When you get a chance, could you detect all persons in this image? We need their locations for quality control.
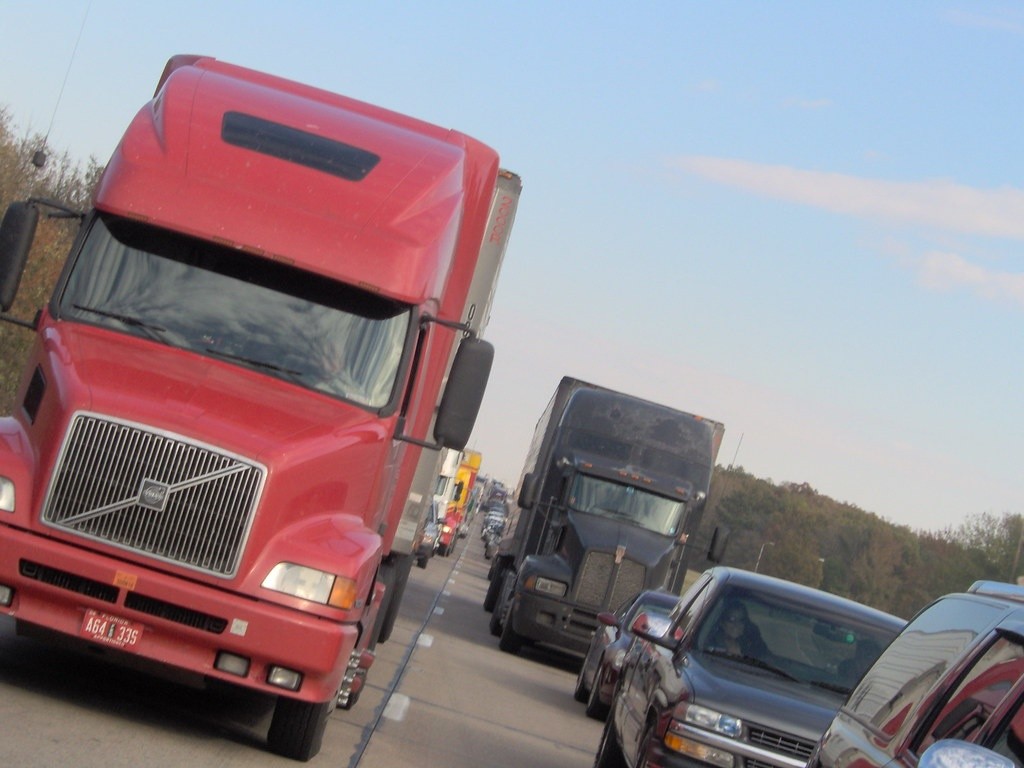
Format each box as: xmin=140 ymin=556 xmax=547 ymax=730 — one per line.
xmin=704 ymin=602 xmax=757 ymax=660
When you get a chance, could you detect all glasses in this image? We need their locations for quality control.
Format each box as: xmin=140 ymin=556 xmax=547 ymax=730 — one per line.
xmin=728 ymin=615 xmax=747 ymax=623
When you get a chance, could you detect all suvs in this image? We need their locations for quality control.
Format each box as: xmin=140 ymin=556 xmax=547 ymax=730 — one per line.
xmin=801 ymin=581 xmax=1024 ymax=768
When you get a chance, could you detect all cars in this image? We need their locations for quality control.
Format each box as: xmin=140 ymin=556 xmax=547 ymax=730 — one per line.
xmin=590 ymin=565 xmax=909 ymax=768
xmin=415 ymin=446 xmax=514 ymax=572
xmin=572 ymin=590 xmax=685 ymax=722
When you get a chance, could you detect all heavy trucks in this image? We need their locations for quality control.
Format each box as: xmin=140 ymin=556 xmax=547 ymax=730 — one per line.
xmin=484 ymin=376 xmax=730 ymax=661
xmin=0 ymin=53 xmax=522 ymax=763
